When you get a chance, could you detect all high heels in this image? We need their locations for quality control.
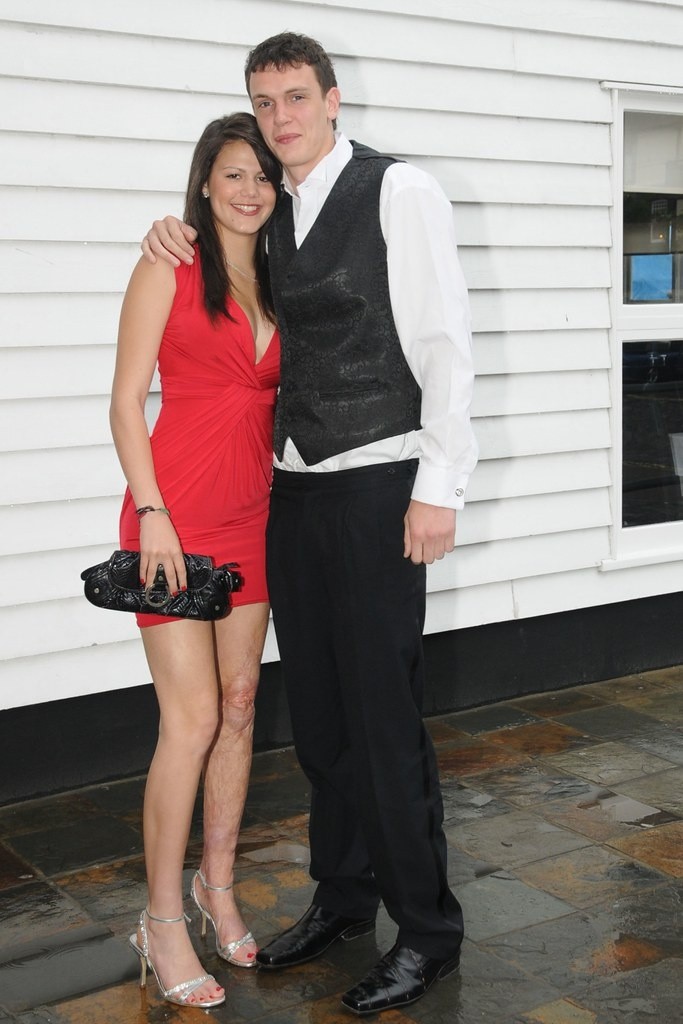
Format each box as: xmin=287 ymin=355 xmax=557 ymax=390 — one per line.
xmin=129 ymin=905 xmax=225 ymax=1008
xmin=189 ymin=868 xmax=261 ymax=968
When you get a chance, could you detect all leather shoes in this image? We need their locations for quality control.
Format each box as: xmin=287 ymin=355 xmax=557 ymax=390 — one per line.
xmin=340 ymin=941 xmax=462 ymax=1015
xmin=255 ymin=904 xmax=377 ymax=969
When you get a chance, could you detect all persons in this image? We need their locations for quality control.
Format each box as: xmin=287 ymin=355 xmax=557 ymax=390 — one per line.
xmin=109 ymin=32 xmax=479 ymax=1014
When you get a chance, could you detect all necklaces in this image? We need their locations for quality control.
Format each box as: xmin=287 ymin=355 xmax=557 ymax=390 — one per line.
xmin=222 ymin=256 xmax=258 ymax=282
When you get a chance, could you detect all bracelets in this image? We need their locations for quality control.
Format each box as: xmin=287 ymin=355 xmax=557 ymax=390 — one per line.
xmin=135 ymin=506 xmax=170 ymax=524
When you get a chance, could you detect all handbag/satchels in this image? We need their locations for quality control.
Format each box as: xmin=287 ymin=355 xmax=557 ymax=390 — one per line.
xmin=80 ymin=547 xmax=242 ymax=622
